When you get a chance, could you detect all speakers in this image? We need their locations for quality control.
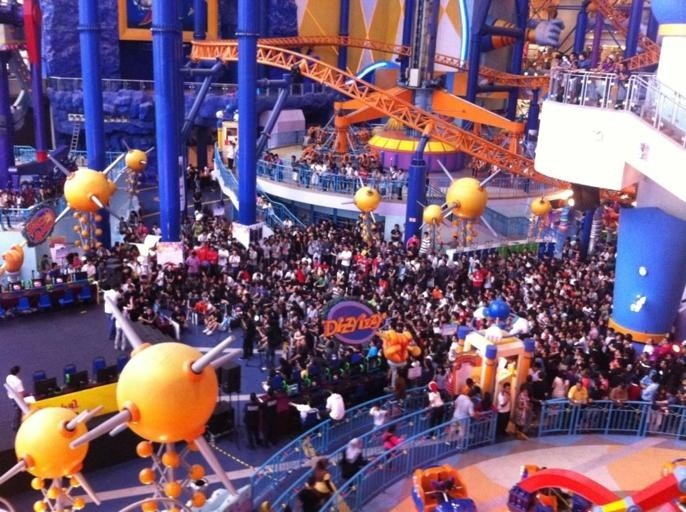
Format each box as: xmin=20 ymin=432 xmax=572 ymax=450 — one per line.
xmin=209 ymin=401 xmax=235 ymax=435
xmin=220 ymin=362 xmax=240 ymax=394
xmin=104 ymin=258 xmax=123 ymax=288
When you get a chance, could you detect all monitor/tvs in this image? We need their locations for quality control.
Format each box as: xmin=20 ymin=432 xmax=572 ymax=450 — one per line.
xmin=70 ymin=370 xmax=88 ymax=385
xmin=36 ymin=377 xmax=57 ymax=393
xmin=97 ymin=365 xmax=117 ymax=381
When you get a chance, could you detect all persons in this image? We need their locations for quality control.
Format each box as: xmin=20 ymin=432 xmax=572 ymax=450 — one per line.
xmin=41 ymin=245 xmax=111 ymax=286
xmin=535 ymin=45 xmax=630 ymax=108
xmin=0 ymin=172 xmax=65 ymax=233
xmin=6 ymin=366 xmax=24 ymax=430
xmin=104 ymin=130 xmax=686 ymax=512
